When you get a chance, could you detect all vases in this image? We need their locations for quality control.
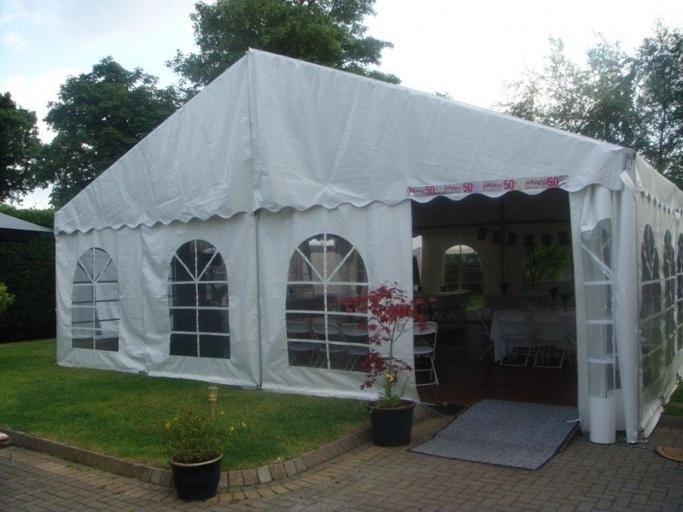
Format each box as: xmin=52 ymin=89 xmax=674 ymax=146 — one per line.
xmin=169 ymin=454 xmax=223 ymax=502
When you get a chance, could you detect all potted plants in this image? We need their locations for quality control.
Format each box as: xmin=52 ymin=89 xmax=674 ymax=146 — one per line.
xmin=342 ymin=281 xmax=437 ymax=447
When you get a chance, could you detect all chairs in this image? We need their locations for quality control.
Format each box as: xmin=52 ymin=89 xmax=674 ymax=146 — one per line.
xmin=287 ymin=320 xmax=438 ymax=388
xmin=476 ymin=306 xmax=569 ymax=371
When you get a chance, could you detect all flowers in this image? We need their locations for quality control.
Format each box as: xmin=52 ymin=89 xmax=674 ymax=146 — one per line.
xmin=165 ymin=403 xmax=245 ymax=460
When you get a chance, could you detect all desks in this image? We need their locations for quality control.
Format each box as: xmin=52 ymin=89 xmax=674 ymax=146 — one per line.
xmin=413 ymin=290 xmax=471 ymax=324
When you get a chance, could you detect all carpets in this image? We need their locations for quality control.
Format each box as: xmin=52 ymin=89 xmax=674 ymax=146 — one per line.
xmin=407 ymin=399 xmax=579 ymax=471
